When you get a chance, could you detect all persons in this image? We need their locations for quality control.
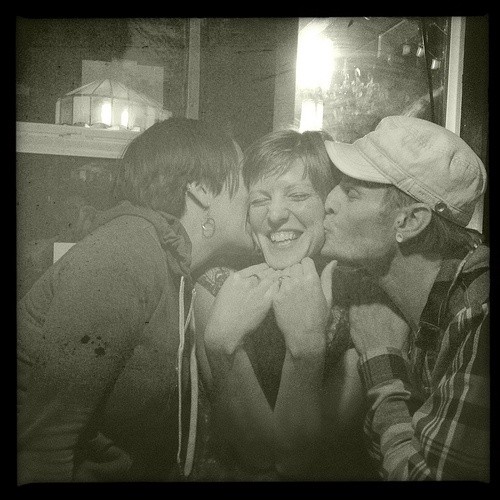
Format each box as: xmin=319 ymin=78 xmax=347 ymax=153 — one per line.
xmin=320 ymin=115 xmax=490 ymax=481
xmin=15 ymin=118 xmax=250 ymax=481
xmin=194 ymin=129 xmax=357 ymax=481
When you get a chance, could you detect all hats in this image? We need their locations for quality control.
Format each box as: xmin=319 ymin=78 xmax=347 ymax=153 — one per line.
xmin=325 ymin=116 xmax=486 ymax=232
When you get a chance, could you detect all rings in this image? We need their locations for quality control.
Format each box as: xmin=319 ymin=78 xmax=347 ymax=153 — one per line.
xmin=278 ymin=274 xmax=292 ymax=283
xmin=248 ymin=274 xmax=261 ymax=286
